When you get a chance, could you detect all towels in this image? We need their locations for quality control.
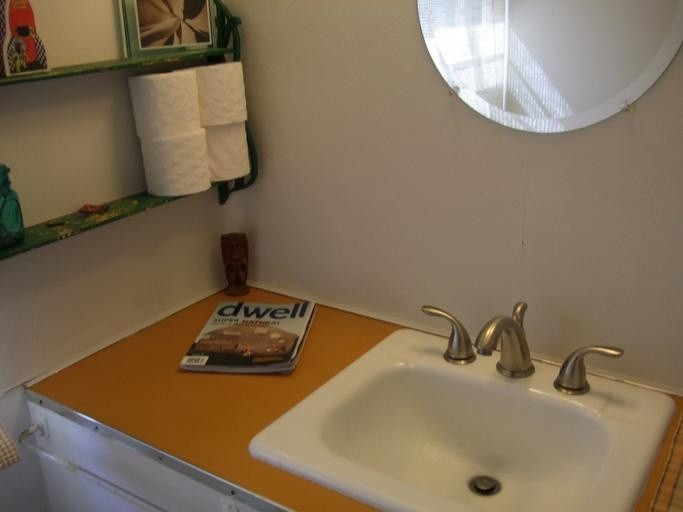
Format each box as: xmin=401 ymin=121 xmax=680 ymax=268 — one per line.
xmin=0 ymin=419 xmax=20 ymax=472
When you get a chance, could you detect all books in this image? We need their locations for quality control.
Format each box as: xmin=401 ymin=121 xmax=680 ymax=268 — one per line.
xmin=177 ymin=300 xmax=319 ymax=377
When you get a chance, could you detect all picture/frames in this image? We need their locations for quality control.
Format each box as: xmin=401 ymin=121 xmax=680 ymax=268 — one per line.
xmin=122 ymin=0 xmax=217 ymax=58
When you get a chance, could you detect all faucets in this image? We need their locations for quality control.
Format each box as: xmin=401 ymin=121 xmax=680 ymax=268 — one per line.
xmin=474 ymin=303 xmax=534 ymax=378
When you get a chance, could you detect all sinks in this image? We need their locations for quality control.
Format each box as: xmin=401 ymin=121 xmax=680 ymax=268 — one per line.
xmin=248 ymin=328 xmax=677 ymax=512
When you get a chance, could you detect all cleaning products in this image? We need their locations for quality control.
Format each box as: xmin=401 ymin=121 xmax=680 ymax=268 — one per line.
xmin=0 ymin=163 xmax=25 ymax=251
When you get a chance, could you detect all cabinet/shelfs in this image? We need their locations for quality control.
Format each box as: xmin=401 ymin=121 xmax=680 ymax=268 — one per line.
xmin=0 ymin=0 xmax=234 ymax=261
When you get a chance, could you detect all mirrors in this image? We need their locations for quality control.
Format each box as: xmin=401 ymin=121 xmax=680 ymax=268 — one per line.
xmin=415 ymin=0 xmax=683 ymax=133
